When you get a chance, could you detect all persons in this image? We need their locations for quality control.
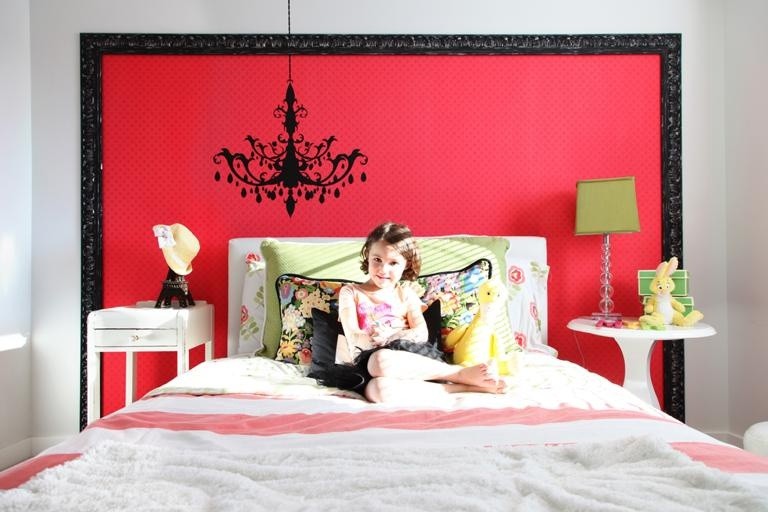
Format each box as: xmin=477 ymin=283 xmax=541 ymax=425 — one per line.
xmin=337 ymin=221 xmax=510 ymax=406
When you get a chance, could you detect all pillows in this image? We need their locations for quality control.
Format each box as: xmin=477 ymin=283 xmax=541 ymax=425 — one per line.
xmin=238 ymin=235 xmax=558 ymax=363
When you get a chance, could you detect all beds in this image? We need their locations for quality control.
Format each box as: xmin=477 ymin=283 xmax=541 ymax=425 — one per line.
xmin=0 ymin=234 xmax=767 ymax=512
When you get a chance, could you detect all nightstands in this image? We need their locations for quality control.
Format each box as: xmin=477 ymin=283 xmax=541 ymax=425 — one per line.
xmin=87 ymin=305 xmax=215 ymax=424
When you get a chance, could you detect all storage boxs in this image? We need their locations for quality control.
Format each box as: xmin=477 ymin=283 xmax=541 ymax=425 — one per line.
xmin=637 ymin=270 xmax=694 ymax=316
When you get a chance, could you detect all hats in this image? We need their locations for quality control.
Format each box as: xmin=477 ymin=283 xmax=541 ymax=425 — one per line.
xmin=153 ymin=223 xmax=201 ymax=276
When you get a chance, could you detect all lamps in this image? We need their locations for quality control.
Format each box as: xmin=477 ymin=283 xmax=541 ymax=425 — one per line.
xmin=575 ymin=176 xmax=640 ymax=321
